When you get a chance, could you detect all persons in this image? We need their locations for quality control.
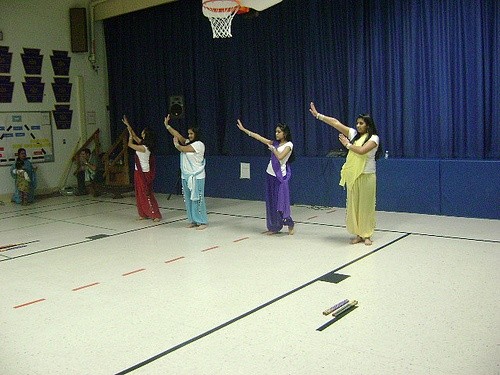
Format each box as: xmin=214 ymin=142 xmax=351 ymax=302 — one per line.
xmin=122 ymin=115 xmax=162 ymax=222
xmin=73 ymin=148 xmax=101 ymax=197
xmin=164 ymin=114 xmax=208 ymax=230
xmin=10 ymin=148 xmax=37 ymax=206
xmin=309 ymin=102 xmax=383 ymax=246
xmin=237 ymin=118 xmax=296 ymax=235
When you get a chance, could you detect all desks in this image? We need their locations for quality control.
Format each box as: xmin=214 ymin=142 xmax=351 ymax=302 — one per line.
xmin=152 ymin=155 xmax=500 ymax=220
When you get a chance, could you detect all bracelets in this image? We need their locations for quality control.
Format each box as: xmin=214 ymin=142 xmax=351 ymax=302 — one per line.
xmin=345 ymin=143 xmax=352 ymax=149
xmin=270 ymin=147 xmax=275 ymax=151
xmin=166 ymin=126 xmax=171 ymax=129
xmin=129 ymin=137 xmax=132 ymax=139
xmin=249 ymin=131 xmax=250 ymax=136
xmin=316 ymin=113 xmax=324 ymax=120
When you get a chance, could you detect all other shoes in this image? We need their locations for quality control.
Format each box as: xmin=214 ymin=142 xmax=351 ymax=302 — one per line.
xmin=76 ymin=190 xmax=88 ymax=196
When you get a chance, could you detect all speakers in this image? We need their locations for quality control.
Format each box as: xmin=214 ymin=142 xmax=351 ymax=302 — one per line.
xmin=69 ymin=8 xmax=88 ymax=52
xmin=168 ymin=96 xmax=185 ymax=119
